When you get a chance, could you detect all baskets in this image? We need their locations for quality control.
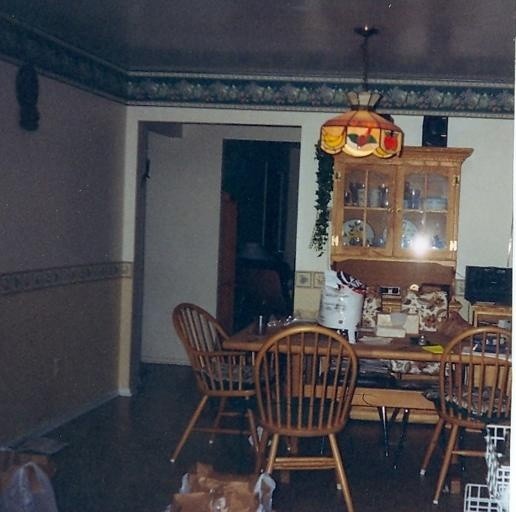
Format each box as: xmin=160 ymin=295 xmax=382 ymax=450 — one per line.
xmin=484 ymin=423 xmax=510 ymax=511
xmin=464 ymin=483 xmax=499 ymax=512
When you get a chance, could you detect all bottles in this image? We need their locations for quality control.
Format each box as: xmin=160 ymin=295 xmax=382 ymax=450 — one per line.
xmin=344 ymin=181 xmax=422 ymax=209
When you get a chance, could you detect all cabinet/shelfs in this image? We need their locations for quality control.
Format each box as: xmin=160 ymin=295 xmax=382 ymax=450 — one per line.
xmin=328 ymin=147 xmax=475 ymax=272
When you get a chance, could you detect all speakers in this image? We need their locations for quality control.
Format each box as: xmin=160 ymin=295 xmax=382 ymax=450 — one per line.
xmin=421 ymin=114 xmax=448 ymax=147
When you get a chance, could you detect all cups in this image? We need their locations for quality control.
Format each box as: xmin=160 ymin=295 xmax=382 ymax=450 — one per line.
xmin=256 ymin=315 xmax=267 ymax=336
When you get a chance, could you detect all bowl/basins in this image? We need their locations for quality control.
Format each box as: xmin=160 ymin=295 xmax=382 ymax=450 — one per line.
xmin=423 ymin=198 xmax=448 ymax=211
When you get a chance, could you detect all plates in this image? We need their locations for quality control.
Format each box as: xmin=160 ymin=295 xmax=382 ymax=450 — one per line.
xmin=341 ymin=218 xmax=376 ymax=248
xmin=382 ymin=219 xmax=419 ymax=252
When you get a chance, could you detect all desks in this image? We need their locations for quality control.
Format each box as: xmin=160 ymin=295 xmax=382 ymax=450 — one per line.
xmin=222 ymin=318 xmax=512 ymax=484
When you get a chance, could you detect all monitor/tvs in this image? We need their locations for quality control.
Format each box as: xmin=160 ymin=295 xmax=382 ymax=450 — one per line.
xmin=462 ymin=264 xmax=511 ymax=305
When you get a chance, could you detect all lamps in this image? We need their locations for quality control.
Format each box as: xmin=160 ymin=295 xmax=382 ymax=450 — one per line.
xmin=319 ymin=26 xmax=404 ymax=159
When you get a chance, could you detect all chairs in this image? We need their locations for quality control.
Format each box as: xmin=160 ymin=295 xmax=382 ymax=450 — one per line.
xmin=251 ymin=321 xmax=360 ymax=512
xmin=419 ymin=325 xmax=511 ymax=505
xmin=469 ymin=303 xmax=511 ymax=330
xmin=169 ymin=302 xmax=274 ymax=473
